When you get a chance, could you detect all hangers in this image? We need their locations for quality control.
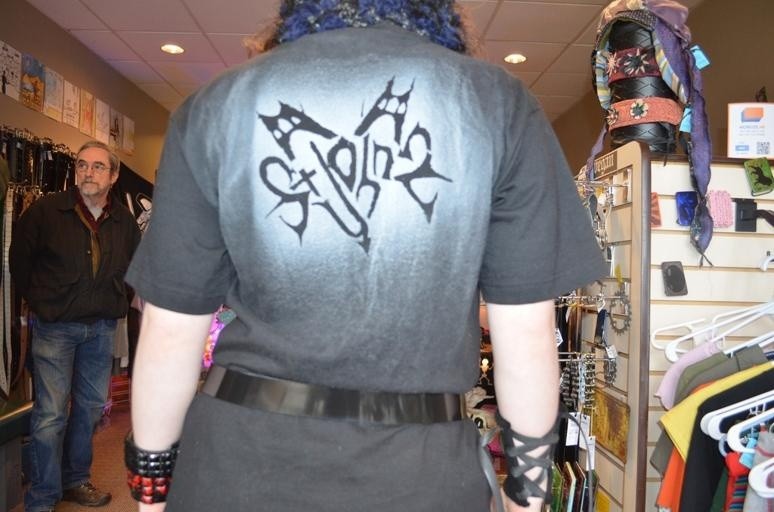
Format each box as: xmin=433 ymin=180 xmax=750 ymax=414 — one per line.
xmin=651 ymin=254 xmax=774 ymax=500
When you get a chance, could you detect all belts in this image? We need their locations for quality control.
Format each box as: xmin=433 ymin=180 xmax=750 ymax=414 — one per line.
xmin=202 ymin=360 xmax=468 ymax=424
xmin=0 ymin=130 xmax=77 ymax=399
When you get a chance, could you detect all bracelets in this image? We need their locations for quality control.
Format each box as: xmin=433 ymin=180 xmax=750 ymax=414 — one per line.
xmin=475 ymin=397 xmax=570 ymax=507
xmin=124 ymin=428 xmax=180 ymax=506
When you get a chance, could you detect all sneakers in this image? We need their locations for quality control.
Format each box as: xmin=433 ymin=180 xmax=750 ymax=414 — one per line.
xmin=67 ymin=483 xmax=111 ymax=508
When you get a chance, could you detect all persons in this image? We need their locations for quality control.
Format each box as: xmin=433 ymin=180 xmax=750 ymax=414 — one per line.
xmin=8 ymin=140 xmax=141 ymax=511
xmin=121 ymin=0 xmax=609 ymax=512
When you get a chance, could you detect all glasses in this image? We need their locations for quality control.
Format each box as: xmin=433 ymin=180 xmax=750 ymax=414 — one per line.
xmin=73 ymin=161 xmax=114 ymax=174
